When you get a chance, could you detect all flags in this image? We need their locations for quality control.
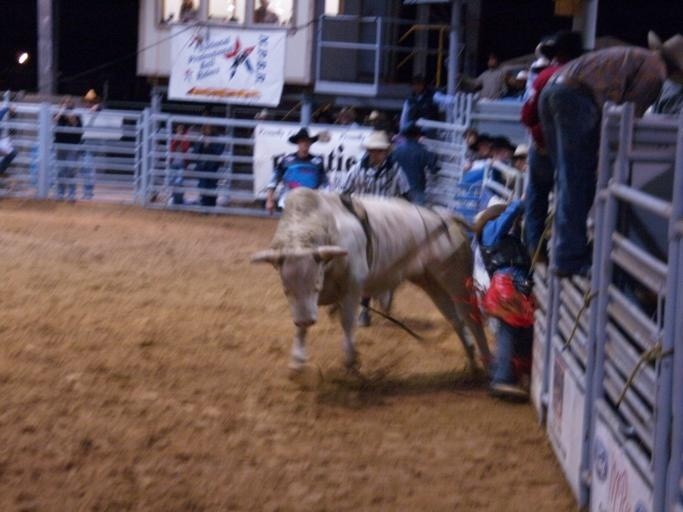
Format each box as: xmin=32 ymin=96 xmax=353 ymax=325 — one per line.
xmin=166 ymin=20 xmax=289 ymax=110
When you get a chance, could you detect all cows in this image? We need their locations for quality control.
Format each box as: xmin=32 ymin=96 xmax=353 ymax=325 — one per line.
xmin=249 ymin=186 xmax=508 ymax=391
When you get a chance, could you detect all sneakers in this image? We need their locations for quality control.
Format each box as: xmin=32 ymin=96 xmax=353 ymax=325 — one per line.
xmin=527 ymin=242 xmax=593 ymax=279
xmin=486 ymin=379 xmax=535 ymax=402
xmin=357 ymin=306 xmax=373 ymax=328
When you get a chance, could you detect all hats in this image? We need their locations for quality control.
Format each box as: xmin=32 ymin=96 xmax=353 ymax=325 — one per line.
xmin=646 ymin=31 xmax=682 ymax=85
xmin=362 ymin=109 xmax=388 ymax=123
xmin=512 ymin=144 xmax=531 ymax=160
xmin=80 ymin=89 xmax=102 ymax=103
xmin=467 ymin=133 xmax=496 ymax=151
xmin=287 ymin=127 xmax=320 ymax=146
xmin=361 ymin=130 xmax=394 ymax=151
xmin=398 ymin=123 xmax=427 ymax=136
xmin=471 ymin=193 xmax=509 ymax=229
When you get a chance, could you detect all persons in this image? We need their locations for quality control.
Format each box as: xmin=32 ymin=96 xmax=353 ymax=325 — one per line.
xmin=178 ymin=0 xmax=199 ymax=21
xmin=252 ymin=0 xmax=279 ymax=24
xmin=471 ymin=191 xmax=540 ymax=403
xmin=192 ymin=107 xmax=226 ymax=206
xmin=47 ymin=93 xmax=85 ymax=205
xmin=72 ymin=87 xmax=104 ymax=201
xmin=163 ymin=120 xmax=195 ymax=204
xmin=0 ymin=86 xmax=28 ymax=175
